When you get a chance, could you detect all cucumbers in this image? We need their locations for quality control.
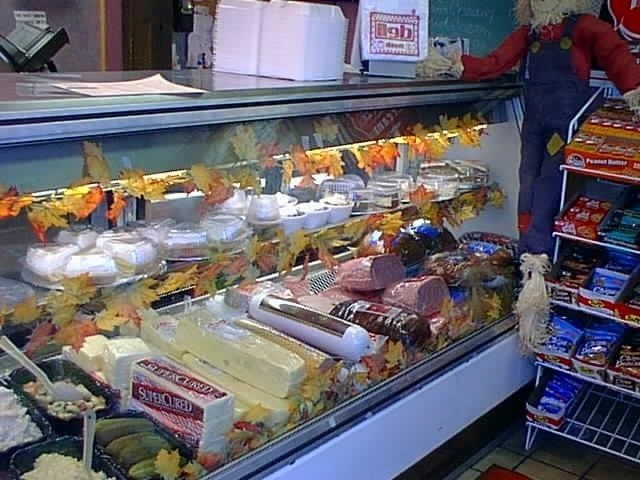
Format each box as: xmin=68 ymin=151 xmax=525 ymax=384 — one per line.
xmin=95 ymin=417 xmax=186 ymax=480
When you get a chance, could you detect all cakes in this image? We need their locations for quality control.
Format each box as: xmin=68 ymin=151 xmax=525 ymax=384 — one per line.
xmin=325 ymin=164 xmax=486 ymax=213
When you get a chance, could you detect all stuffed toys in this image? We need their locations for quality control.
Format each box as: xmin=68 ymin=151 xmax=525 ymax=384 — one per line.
xmin=413 ymin=0 xmax=639 ymax=353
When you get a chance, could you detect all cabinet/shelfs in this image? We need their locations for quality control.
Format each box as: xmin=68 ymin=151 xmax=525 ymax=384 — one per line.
xmin=2 ymin=68 xmax=541 ymax=478
xmin=524 ymin=85 xmax=640 ymax=462
